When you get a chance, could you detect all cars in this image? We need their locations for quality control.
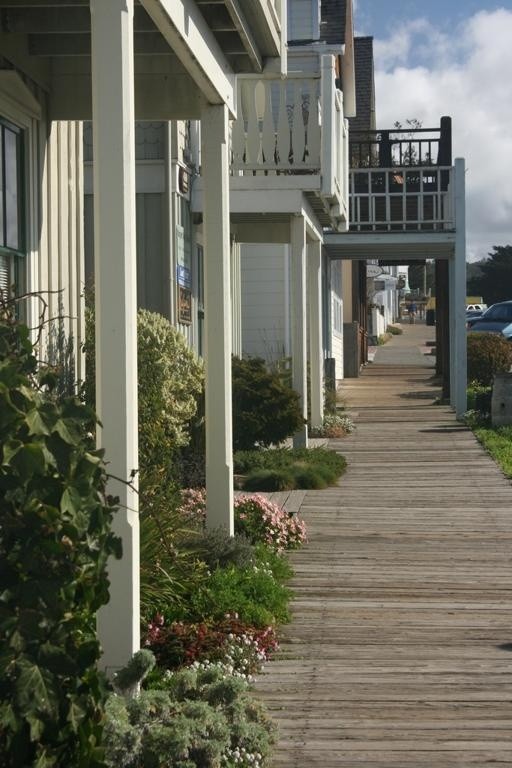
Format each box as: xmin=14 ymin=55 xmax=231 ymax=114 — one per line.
xmin=466 ymin=300 xmax=512 ymax=341
